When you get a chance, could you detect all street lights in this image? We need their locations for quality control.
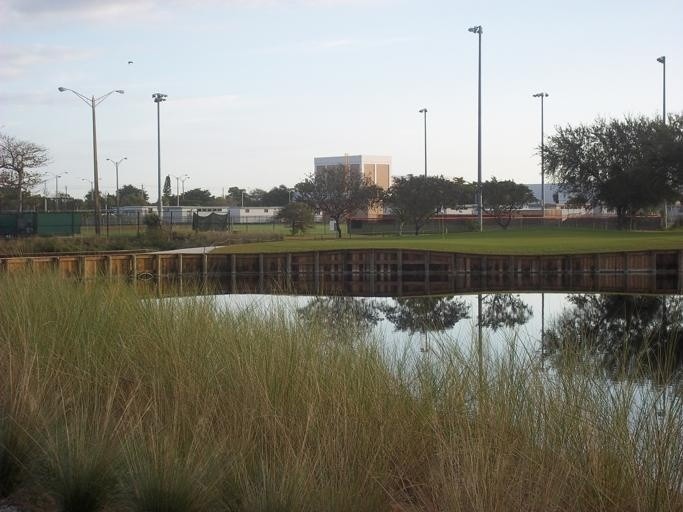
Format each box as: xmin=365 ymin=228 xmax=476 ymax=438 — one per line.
xmin=418 ymin=108 xmax=429 ymax=179
xmin=531 ymin=92 xmax=551 ymax=209
xmin=467 ymin=23 xmax=486 ymax=232
xmin=151 ymin=90 xmax=170 ymax=222
xmin=46 ymin=170 xmax=69 ymax=209
xmin=169 ymin=172 xmax=188 ymax=206
xmin=654 ymin=55 xmax=666 ymax=125
xmin=104 ymin=156 xmax=127 ymax=207
xmin=55 ymin=85 xmax=126 ymax=236
xmin=80 ymin=175 xmax=102 ymax=208
